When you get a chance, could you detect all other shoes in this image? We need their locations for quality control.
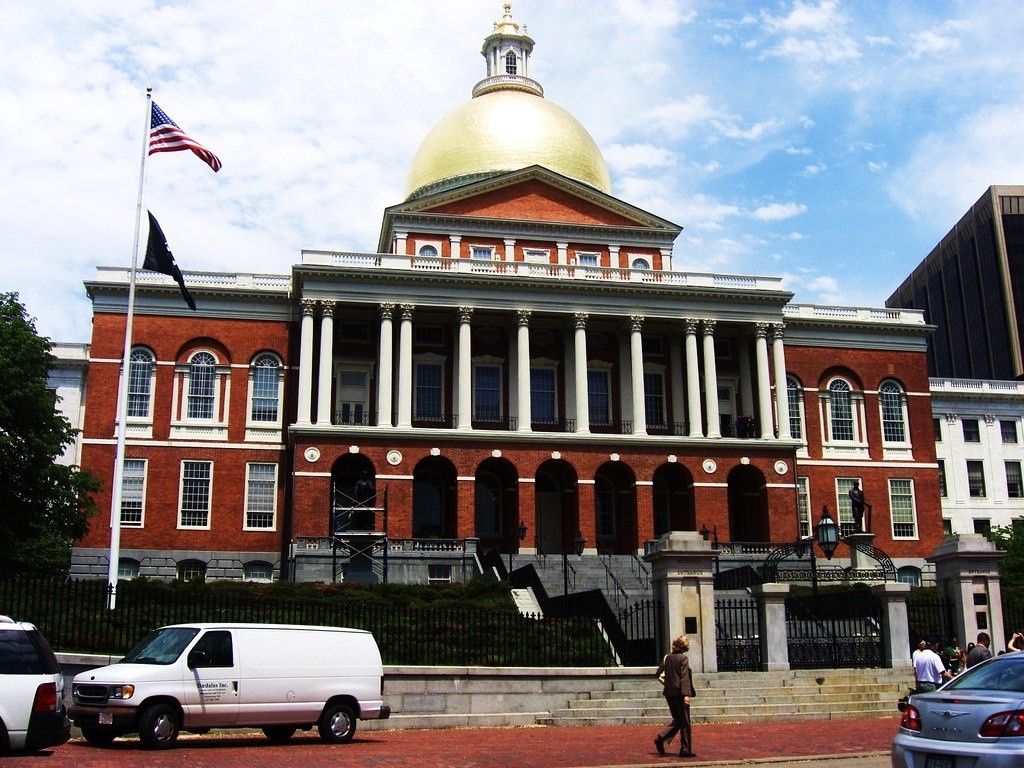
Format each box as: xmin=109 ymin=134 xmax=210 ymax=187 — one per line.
xmin=654 ymin=736 xmax=667 ymax=754
xmin=681 ymin=752 xmax=697 ymax=758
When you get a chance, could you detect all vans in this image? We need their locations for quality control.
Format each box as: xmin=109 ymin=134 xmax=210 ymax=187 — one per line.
xmin=66 ymin=622 xmax=393 ymax=752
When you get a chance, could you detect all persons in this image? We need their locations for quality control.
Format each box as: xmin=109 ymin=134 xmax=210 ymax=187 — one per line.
xmin=654 ymin=635 xmax=697 ymax=756
xmin=911 ymin=632 xmax=1024 ymax=692
xmin=849 ymin=481 xmax=871 ymax=532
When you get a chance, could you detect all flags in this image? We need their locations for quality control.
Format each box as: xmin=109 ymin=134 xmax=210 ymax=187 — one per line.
xmin=148 ymin=101 xmax=222 ymax=172
xmin=142 ymin=210 xmax=196 ymax=312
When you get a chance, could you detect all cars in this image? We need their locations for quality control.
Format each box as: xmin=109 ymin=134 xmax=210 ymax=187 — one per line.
xmin=0 ymin=613 xmax=71 ymax=755
xmin=891 ymin=646 xmax=1024 ymax=768
